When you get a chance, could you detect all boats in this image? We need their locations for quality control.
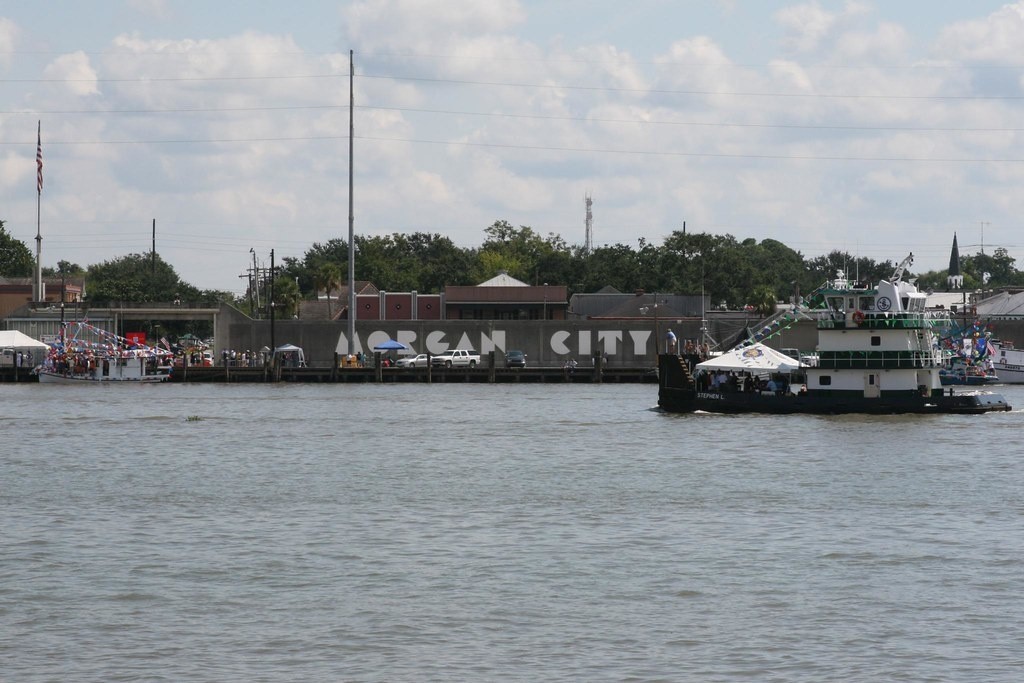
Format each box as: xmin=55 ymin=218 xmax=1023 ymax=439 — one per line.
xmin=657 ymin=252 xmax=1013 ymax=415
xmin=937 ymin=292 xmax=1000 ymax=385
xmin=38 ymin=311 xmax=175 ymax=384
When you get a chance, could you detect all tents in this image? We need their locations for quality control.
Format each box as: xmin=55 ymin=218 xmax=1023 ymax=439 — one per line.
xmin=374 ymin=340 xmax=409 ymax=367
xmin=695 ymin=342 xmax=812 ymax=396
xmin=273 ymin=344 xmax=306 ymax=368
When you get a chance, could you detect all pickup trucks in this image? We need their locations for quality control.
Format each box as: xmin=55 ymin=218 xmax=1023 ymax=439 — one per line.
xmin=433 ymin=349 xmax=480 ymax=368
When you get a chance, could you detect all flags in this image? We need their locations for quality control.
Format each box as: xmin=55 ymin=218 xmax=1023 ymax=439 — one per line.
xmin=986 ymin=339 xmax=996 ymax=354
xmin=36 ymin=122 xmax=43 ymax=193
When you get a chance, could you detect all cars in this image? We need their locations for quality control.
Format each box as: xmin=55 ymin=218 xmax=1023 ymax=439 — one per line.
xmin=396 ymin=353 xmax=433 ymax=368
xmin=196 ymin=352 xmax=214 ymax=365
xmin=505 ymin=351 xmax=527 ymax=366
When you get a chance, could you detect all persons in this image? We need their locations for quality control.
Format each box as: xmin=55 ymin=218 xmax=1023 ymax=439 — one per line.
xmin=684 ymin=340 xmax=710 ymax=361
xmin=346 ymin=352 xmax=351 ymax=367
xmin=666 ymin=329 xmax=676 ymax=354
xmin=570 ymin=358 xmax=577 ymax=368
xmin=710 ymin=371 xmax=777 ymax=396
xmin=66 ymin=358 xmax=75 ymax=377
xmin=81 ymin=357 xmax=90 ymax=374
xmin=26 ymin=351 xmax=33 ymax=367
xmin=221 ymin=347 xmax=264 ymax=367
xmin=17 ymin=351 xmax=23 ymax=367
xmin=190 ymin=351 xmax=205 ymax=366
xmin=988 ymin=358 xmax=994 ymax=375
xmin=286 ymin=353 xmax=293 ymax=359
xmin=356 ymin=352 xmax=366 ymax=367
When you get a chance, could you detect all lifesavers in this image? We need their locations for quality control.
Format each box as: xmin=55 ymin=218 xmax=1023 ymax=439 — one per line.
xmin=852 ymin=311 xmax=865 ymax=324
